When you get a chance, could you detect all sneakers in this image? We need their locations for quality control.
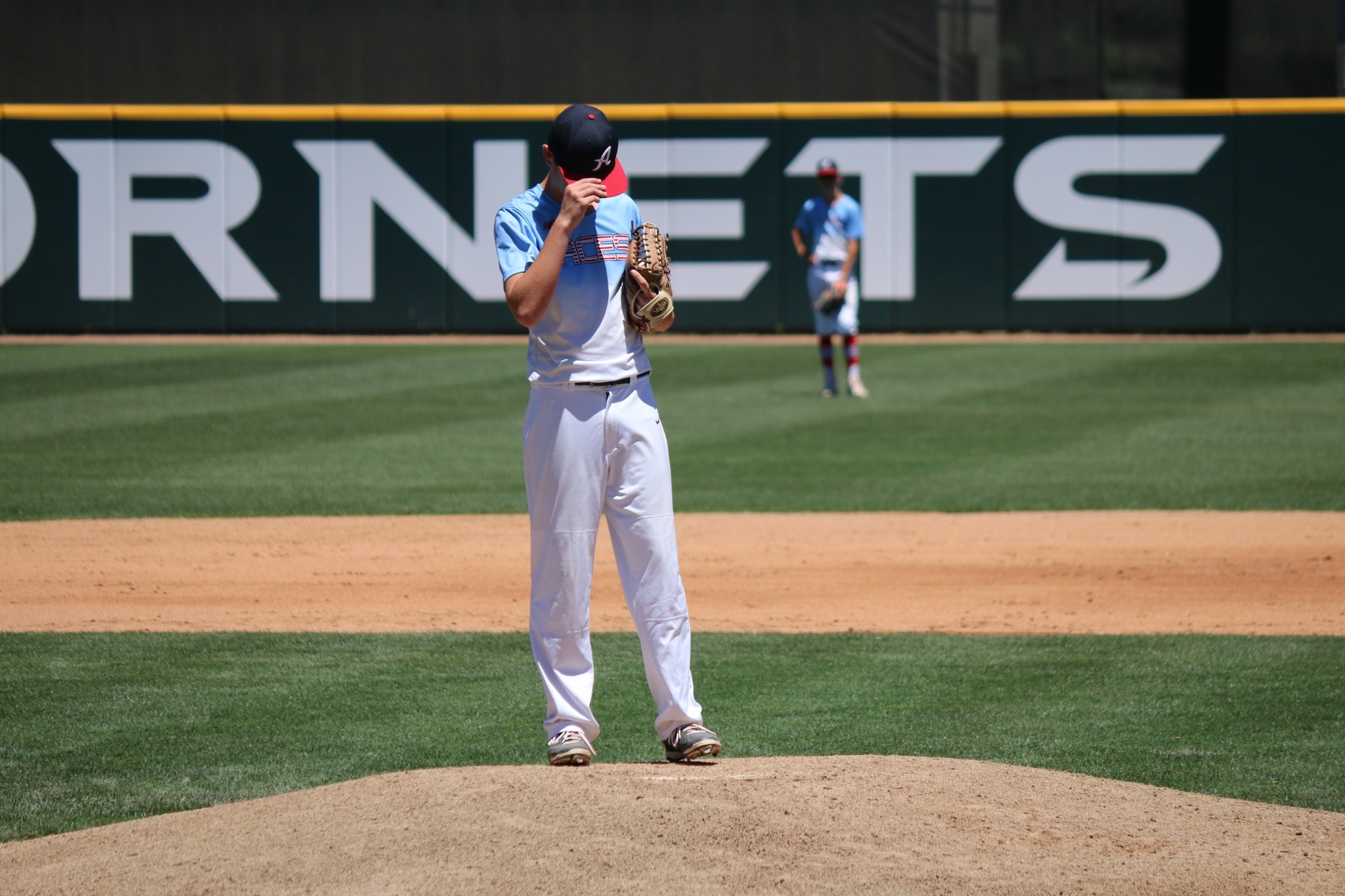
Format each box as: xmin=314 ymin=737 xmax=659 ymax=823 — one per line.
xmin=662 ymin=723 xmax=721 ymax=761
xmin=548 ymin=731 xmax=591 ymax=764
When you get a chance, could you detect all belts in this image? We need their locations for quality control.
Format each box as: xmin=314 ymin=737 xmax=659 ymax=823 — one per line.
xmin=575 ymin=370 xmax=650 ymax=387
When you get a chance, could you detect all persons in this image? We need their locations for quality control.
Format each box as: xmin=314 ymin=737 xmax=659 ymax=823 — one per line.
xmin=495 ymin=103 xmax=719 ymax=763
xmin=791 ymin=158 xmax=868 ymax=399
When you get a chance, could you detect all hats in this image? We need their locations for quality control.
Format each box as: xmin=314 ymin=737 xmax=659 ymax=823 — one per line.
xmin=818 ymin=158 xmax=837 ymax=177
xmin=549 ymin=105 xmax=627 ymax=198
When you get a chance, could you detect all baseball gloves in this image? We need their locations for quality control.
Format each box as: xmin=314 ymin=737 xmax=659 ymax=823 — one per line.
xmin=814 ymin=286 xmax=844 ymax=315
xmin=624 ymin=222 xmax=675 ymax=333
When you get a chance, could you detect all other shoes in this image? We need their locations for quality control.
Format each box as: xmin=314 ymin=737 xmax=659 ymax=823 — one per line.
xmin=847 ymin=380 xmax=869 ymax=398
xmin=822 ymin=383 xmax=837 ymax=398
